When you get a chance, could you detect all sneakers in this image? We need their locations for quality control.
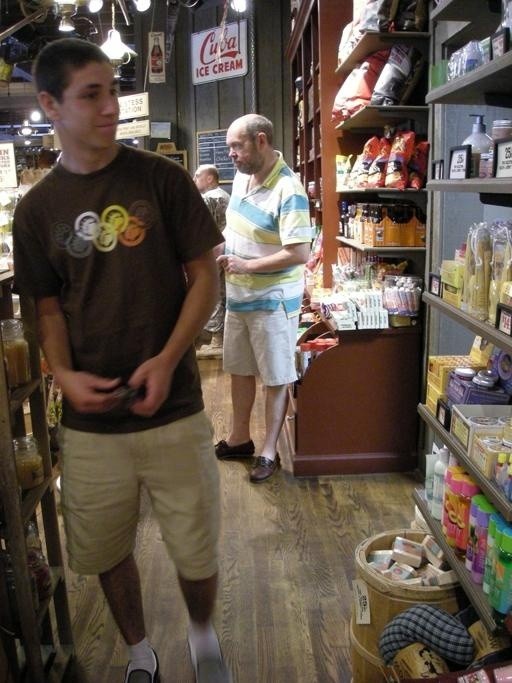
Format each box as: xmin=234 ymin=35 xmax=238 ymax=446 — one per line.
xmin=124 ymin=648 xmax=159 ymax=682
xmin=187 ymin=629 xmax=226 ymax=682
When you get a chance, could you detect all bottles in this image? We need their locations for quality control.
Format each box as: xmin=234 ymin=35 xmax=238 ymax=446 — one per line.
xmin=0 ymin=319 xmax=32 ymax=387
xmin=0 ymin=520 xmax=53 ymax=619
xmin=12 ymin=436 xmax=44 ymax=490
xmin=337 ymin=201 xmax=410 ymax=244
xmin=151 ymin=37 xmax=164 ymax=74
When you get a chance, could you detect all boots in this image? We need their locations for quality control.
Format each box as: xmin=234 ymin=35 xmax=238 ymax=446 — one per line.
xmin=201 ymin=344 xmax=209 ymax=350
xmin=195 ymin=332 xmax=223 ymax=360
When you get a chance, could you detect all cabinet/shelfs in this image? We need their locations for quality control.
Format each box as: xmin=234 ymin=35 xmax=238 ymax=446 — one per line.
xmin=412 ymin=1 xmax=512 ymax=637
xmin=0 ymin=270 xmax=77 ymax=683
xmin=283 ymin=0 xmax=430 ymax=475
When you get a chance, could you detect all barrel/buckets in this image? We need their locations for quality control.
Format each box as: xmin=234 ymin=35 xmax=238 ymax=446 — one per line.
xmin=349 ymin=527 xmax=464 ymax=683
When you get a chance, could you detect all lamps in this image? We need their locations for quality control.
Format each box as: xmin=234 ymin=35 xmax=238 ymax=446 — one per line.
xmin=95 ymin=0 xmax=138 ymax=81
xmin=52 ymin=0 xmax=78 ymax=32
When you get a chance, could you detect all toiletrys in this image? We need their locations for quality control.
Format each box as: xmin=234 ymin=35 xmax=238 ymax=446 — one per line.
xmin=424 ymin=440 xmax=435 ymax=506
xmin=461 ymin=113 xmax=491 ymax=178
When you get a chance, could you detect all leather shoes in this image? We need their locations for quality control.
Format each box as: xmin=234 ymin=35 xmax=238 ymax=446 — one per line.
xmin=214 ymin=441 xmax=254 ymax=459
xmin=250 ymin=453 xmax=280 ymax=482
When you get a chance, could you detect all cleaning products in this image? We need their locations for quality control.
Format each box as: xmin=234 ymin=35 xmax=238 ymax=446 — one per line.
xmin=432 ymin=444 xmax=459 ymax=520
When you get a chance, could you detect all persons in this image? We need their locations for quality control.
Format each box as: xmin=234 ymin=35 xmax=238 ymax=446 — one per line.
xmin=194 ymin=162 xmax=231 ymax=361
xmin=13 ymin=36 xmax=227 ymax=683
xmin=212 ymin=111 xmax=313 ymax=483
